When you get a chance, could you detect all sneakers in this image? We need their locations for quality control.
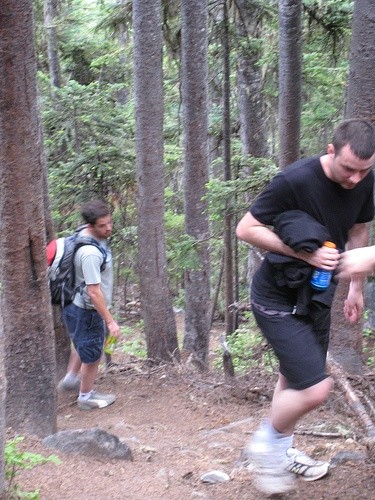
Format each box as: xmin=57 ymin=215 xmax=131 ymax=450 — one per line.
xmin=77 ymin=390 xmax=116 ymax=410
xmin=56 ymin=377 xmax=81 ymax=394
xmin=244 ymin=423 xmax=330 ymax=495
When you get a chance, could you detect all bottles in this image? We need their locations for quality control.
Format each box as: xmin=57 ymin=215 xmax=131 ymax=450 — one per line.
xmin=310 ymin=241 xmax=336 ymax=292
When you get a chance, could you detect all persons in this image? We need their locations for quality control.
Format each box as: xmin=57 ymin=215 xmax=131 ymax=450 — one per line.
xmin=332 ymin=240 xmax=375 ymax=283
xmin=56 ymin=200 xmax=121 ymax=411
xmin=235 ymin=117 xmax=375 ymax=498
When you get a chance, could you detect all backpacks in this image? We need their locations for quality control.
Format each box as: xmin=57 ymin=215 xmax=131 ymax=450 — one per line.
xmin=44 ymin=224 xmax=107 ymax=309
